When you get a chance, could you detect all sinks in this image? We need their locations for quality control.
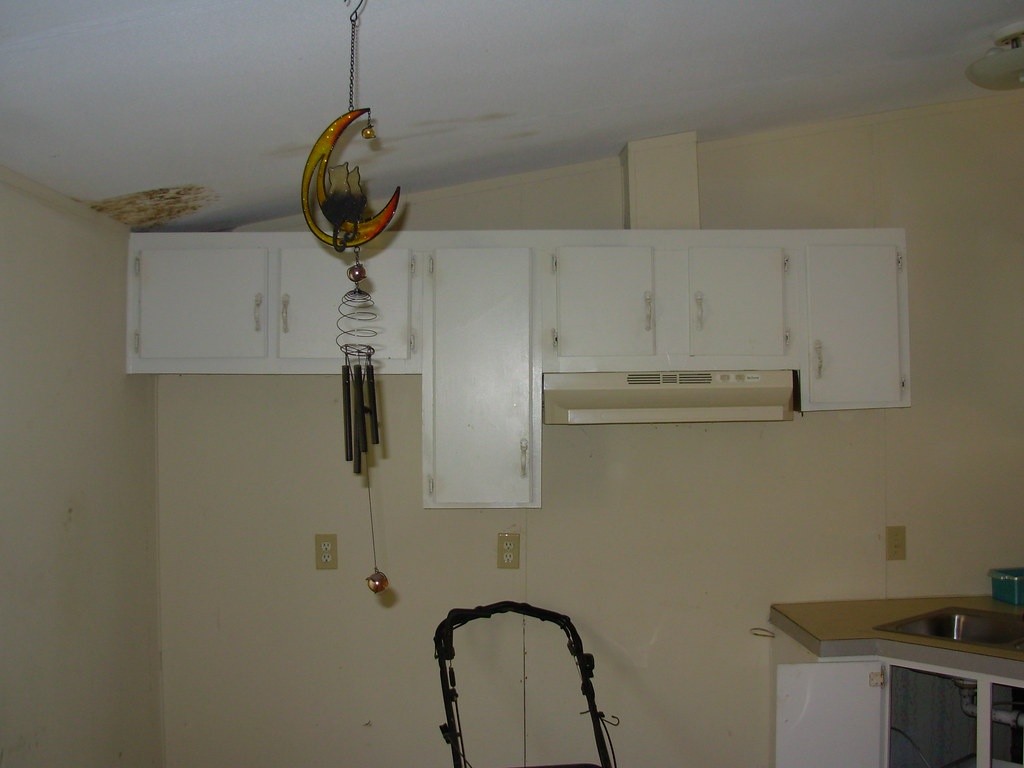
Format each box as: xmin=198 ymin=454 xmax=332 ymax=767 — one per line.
xmin=871 ymin=605 xmax=1024 ymax=652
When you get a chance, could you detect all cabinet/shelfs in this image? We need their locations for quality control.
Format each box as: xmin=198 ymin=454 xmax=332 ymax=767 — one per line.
xmin=801 ymin=228 xmax=911 ymax=411
xmin=422 ymin=231 xmax=543 ymax=512
xmin=125 ymin=233 xmax=422 ymax=375
xmin=543 ymin=230 xmax=801 ymax=371
xmin=776 ymin=655 xmax=1024 ymax=768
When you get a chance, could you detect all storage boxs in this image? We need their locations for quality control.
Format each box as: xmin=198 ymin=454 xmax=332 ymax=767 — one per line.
xmin=989 ymin=567 xmax=1024 ymax=605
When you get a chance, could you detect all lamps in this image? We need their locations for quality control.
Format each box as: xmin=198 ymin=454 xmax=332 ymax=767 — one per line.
xmin=965 ymin=22 xmax=1024 ymax=91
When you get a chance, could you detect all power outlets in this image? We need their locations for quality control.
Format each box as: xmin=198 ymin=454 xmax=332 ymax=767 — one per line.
xmin=315 ymin=534 xmax=338 ymax=569
xmin=886 ymin=526 xmax=906 ymax=560
xmin=497 ymin=534 xmax=520 ymax=568
